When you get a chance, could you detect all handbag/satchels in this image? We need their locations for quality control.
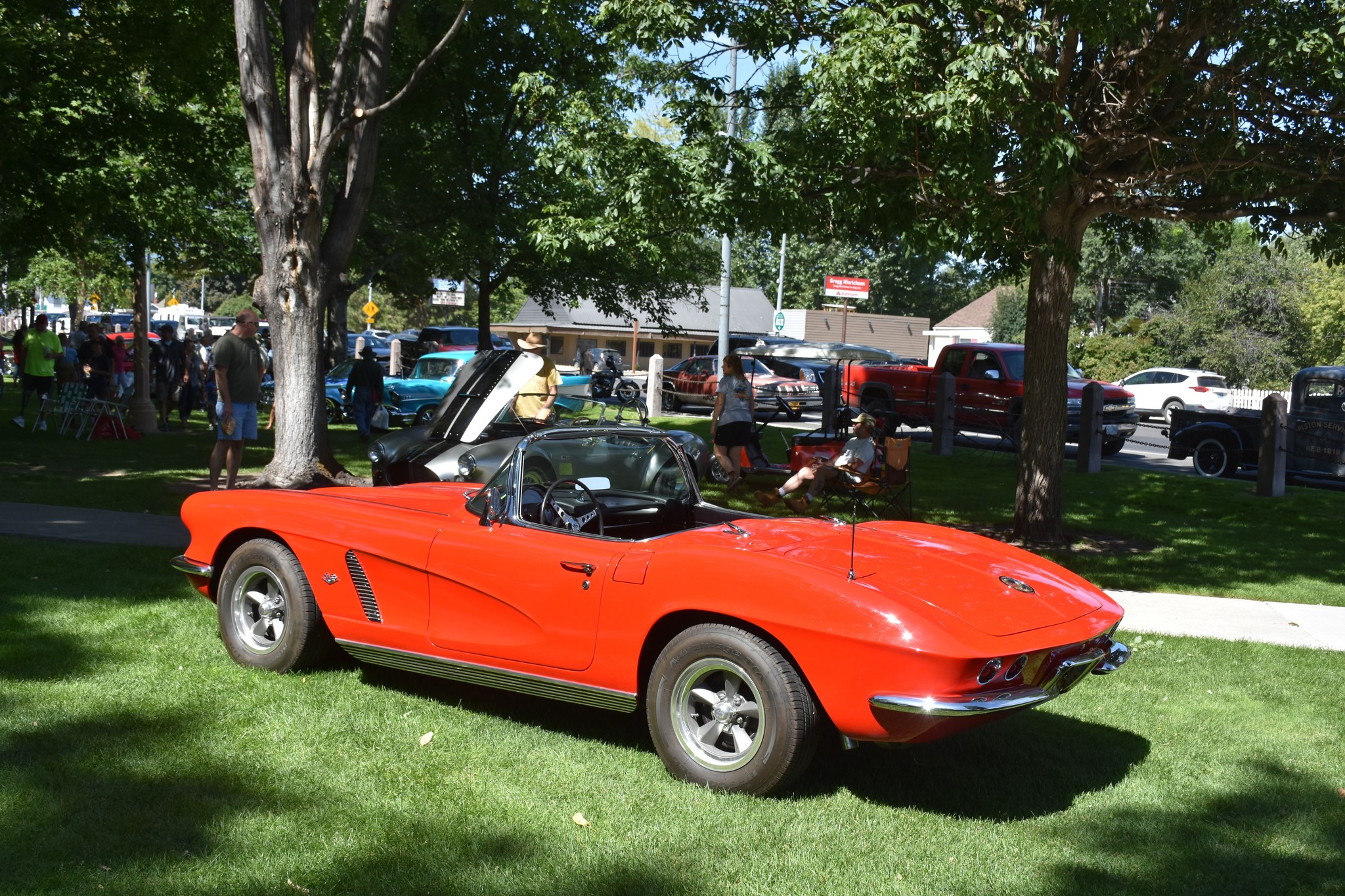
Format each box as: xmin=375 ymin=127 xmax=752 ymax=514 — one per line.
xmin=370 ymin=404 xmax=389 ymax=430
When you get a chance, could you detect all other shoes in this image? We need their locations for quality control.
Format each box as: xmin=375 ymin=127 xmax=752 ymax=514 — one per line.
xmin=724 ymin=474 xmax=742 ymax=493
xmin=363 ymin=434 xmax=369 ymax=444
xmin=12 ymin=417 xmax=24 ymax=429
xmin=39 ymin=424 xmax=48 ymax=431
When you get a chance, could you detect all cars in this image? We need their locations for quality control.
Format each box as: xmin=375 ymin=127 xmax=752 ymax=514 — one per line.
xmin=642 ymin=354 xmax=823 ymax=422
xmin=205 ymin=326 xmax=514 ymax=424
xmin=6 ymin=306 xmax=272 ymax=394
xmin=573 ymin=348 xmax=624 ymax=374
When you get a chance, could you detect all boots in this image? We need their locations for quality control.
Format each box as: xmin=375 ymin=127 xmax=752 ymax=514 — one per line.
xmin=754 ymin=487 xmax=780 ymax=508
xmin=785 ymin=494 xmax=809 ymax=515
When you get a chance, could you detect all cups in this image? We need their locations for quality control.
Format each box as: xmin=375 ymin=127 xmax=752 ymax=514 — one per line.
xmin=45 ymin=347 xmax=51 ymax=360
xmin=83 ymin=366 xmax=91 ymax=379
xmin=821 ymin=455 xmax=829 ymax=463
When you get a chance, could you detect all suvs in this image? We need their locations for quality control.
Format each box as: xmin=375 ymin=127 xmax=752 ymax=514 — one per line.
xmin=1111 ymin=367 xmax=1233 ymax=425
xmin=707 ymin=334 xmax=924 ymax=387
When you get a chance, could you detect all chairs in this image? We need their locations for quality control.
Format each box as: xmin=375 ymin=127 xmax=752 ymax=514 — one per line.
xmin=969 ymin=359 xmax=988 ymax=378
xmin=811 ymin=435 xmax=912 ymax=521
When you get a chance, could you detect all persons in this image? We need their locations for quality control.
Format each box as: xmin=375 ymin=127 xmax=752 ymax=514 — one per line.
xmin=345 ymin=346 xmax=384 ymax=444
xmin=509 ymin=333 xmax=562 ymax=427
xmin=753 ymin=413 xmax=878 ymax=513
xmin=0 ymin=334 xmax=5 ymax=401
xmin=578 ymin=346 xmax=594 ymax=376
xmin=210 ymin=309 xmax=263 ymax=493
xmin=12 ymin=314 xmax=276 ymax=433
xmin=711 ymin=355 xmax=756 ymax=494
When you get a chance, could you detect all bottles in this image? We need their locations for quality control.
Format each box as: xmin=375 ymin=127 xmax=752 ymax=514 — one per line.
xmin=880 ymin=463 xmax=885 ymax=478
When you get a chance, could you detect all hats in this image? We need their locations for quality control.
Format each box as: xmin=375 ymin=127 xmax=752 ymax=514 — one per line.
xmin=36 ymin=314 xmax=48 ymax=322
xmin=158 ymin=324 xmax=174 ymax=332
xmin=517 ymin=332 xmax=549 ymax=349
xmin=851 ymin=412 xmax=876 ymax=427
xmin=358 ymin=346 xmax=377 ymax=357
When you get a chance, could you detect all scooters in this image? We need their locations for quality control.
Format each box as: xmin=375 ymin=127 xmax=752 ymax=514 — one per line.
xmin=708 ymin=395 xmax=904 ymax=495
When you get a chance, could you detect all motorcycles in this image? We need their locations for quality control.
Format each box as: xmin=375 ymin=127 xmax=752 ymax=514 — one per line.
xmin=560 ymin=350 xmax=640 ymax=405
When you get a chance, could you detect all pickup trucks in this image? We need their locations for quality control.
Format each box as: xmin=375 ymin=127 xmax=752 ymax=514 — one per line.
xmin=1161 ymin=365 xmax=1345 ymax=480
xmin=840 ymin=342 xmax=1139 ymax=457
xmin=373 ymin=349 xmax=592 ymax=429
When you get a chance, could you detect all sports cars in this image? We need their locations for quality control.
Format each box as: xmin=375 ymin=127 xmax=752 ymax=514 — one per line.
xmin=170 ymin=422 xmax=1131 ymax=799
xmin=367 ymin=349 xmax=706 ymax=499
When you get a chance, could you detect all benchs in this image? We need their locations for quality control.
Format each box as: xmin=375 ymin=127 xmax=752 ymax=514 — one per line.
xmin=791 ymin=407 xmax=851 ymax=446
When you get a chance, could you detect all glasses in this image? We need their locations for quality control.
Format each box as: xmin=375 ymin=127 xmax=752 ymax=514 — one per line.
xmin=242 ymin=321 xmax=259 ymax=327
